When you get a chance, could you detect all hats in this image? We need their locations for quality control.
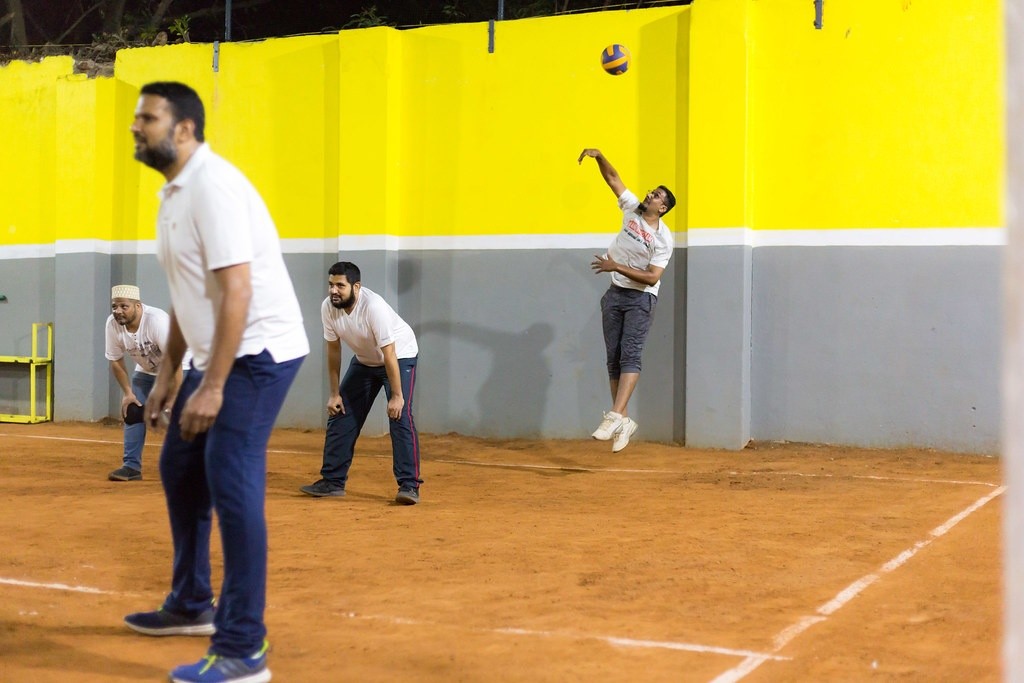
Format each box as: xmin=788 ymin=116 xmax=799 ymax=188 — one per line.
xmin=111 ymin=284 xmax=142 ymax=302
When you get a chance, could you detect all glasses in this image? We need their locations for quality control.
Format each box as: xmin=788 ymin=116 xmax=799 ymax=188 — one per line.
xmin=648 ymin=189 xmax=669 ymax=209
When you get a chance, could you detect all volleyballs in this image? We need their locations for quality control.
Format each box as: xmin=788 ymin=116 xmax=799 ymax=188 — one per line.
xmin=600 ymin=44 xmax=633 ymax=75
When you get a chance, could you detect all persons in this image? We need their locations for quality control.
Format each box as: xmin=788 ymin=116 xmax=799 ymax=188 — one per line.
xmin=298 ymin=262 xmax=422 ymax=506
xmin=123 ymin=81 xmax=310 ymax=683
xmin=578 ymin=148 xmax=677 ymax=453
xmin=106 ymin=285 xmax=192 ymax=487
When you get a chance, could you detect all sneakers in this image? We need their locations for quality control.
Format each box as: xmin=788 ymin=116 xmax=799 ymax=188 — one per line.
xmin=122 ymin=597 xmax=221 ymax=638
xmin=298 ymin=478 xmax=346 ymax=497
xmin=395 ymin=482 xmax=420 ymax=504
xmin=592 ymin=411 xmax=624 ymax=441
xmin=107 ymin=465 xmax=144 ymax=482
xmin=611 ymin=417 xmax=639 ymax=454
xmin=167 ymin=638 xmax=274 ymax=683
